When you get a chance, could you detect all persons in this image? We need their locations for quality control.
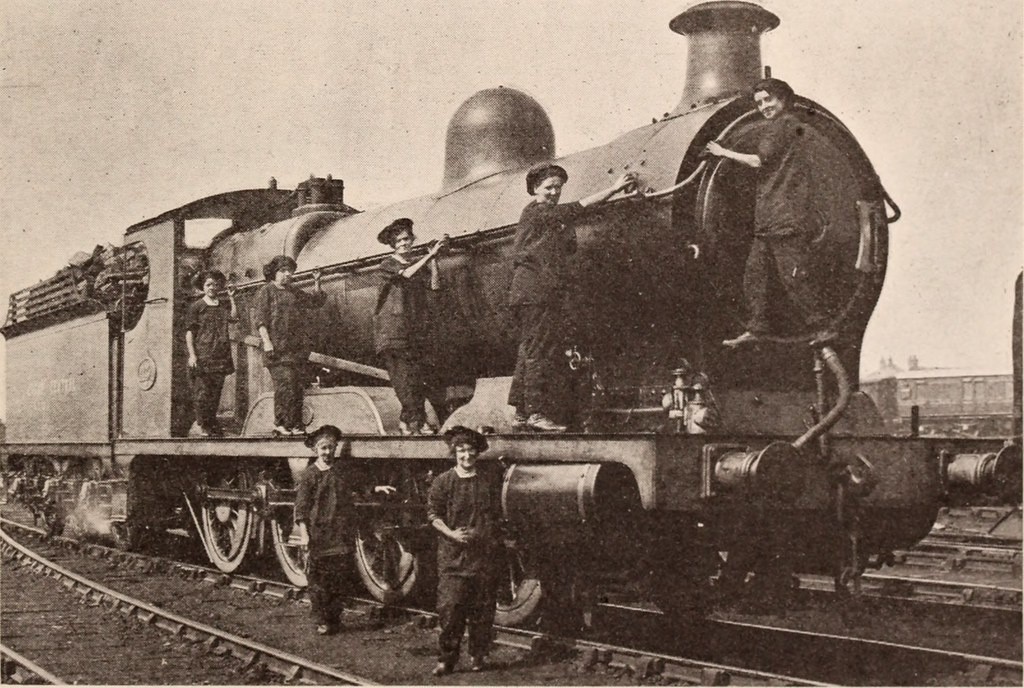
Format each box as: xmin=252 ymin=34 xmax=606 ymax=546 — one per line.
xmin=371 ymin=217 xmax=447 ymax=436
xmin=185 ymin=269 xmax=240 ymax=438
xmin=508 ymin=162 xmax=634 ymax=433
xmin=294 ymin=425 xmax=357 ymax=634
xmin=254 ymin=254 xmax=327 ymax=436
xmin=426 ymin=426 xmax=501 ymax=674
xmin=706 ymin=79 xmax=836 ymax=346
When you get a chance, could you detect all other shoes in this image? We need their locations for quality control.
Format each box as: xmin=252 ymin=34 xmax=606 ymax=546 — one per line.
xmin=471 ymin=656 xmax=484 ymax=672
xmin=313 ymin=624 xmax=340 ymax=635
xmin=398 ymin=421 xmax=438 ymax=435
xmin=273 ymin=426 xmax=306 ymax=435
xmin=432 ymin=663 xmax=454 ymax=676
xmin=514 ymin=413 xmax=566 ymax=432
xmin=189 ymin=422 xmax=224 ymax=436
xmin=722 ymin=332 xmax=764 ymax=348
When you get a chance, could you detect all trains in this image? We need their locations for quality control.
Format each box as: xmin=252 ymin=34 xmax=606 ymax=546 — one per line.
xmin=0 ymin=0 xmax=941 ymax=628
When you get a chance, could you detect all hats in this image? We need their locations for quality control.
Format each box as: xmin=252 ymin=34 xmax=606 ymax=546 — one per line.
xmin=304 ymin=425 xmax=343 ymax=447
xmin=191 ymin=269 xmax=228 ymax=291
xmin=527 ymin=161 xmax=568 ymax=196
xmin=440 ymin=425 xmax=489 ymax=453
xmin=264 ymin=255 xmax=297 ymax=281
xmin=377 ymin=218 xmax=413 ymax=245
